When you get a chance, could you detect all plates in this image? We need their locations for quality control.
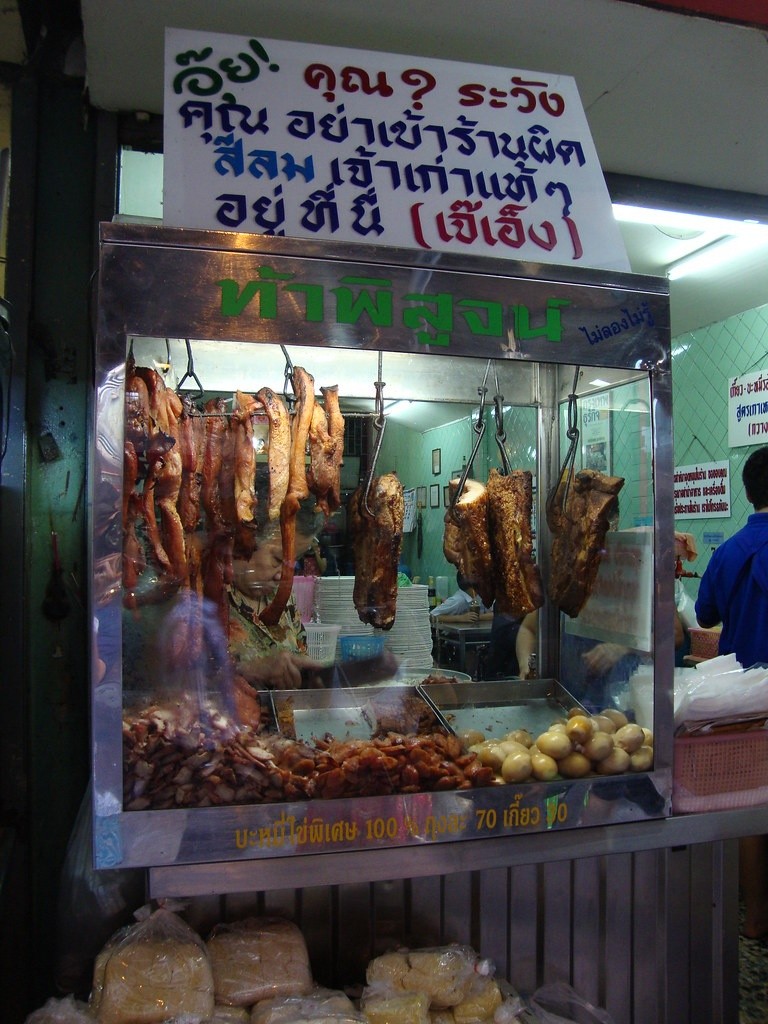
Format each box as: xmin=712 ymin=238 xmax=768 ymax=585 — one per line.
xmin=316 ymin=576 xmax=375 ymax=656
xmin=375 ymin=584 xmax=433 ymax=669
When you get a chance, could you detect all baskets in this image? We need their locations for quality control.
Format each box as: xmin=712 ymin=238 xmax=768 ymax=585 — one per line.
xmin=290 ymin=573 xmax=315 ymax=623
xmin=341 ymin=631 xmax=386 ymax=675
xmin=300 ymin=622 xmax=342 ymax=667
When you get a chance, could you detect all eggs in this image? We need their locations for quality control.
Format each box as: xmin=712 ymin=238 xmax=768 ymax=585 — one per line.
xmin=461 ymin=707 xmax=653 ymax=785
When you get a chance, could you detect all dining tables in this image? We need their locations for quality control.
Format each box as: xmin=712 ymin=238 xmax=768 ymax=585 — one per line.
xmin=436 ymin=621 xmax=493 ymax=673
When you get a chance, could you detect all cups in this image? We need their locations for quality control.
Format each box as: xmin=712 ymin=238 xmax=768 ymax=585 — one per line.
xmin=469 ymin=601 xmax=480 ymax=622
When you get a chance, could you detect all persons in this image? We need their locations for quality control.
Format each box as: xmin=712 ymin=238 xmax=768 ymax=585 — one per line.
xmin=294 ymin=539 xmax=334 ymax=577
xmin=491 ymin=608 xmax=642 ymax=715
xmin=695 ymin=448 xmax=768 ymax=673
xmin=166 ymin=494 xmax=320 ymax=726
xmin=430 ymin=571 xmax=493 ymax=622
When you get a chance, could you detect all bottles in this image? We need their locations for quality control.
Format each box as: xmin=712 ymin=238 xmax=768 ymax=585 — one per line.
xmin=427 ymin=576 xmax=449 ymax=610
xmin=414 ymin=576 xmax=420 ymax=584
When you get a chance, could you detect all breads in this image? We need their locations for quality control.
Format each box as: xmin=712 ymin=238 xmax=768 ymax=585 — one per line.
xmin=29 ymin=920 xmax=518 ymax=1024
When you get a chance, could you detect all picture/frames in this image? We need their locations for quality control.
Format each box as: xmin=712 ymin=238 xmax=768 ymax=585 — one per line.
xmin=452 ymin=469 xmax=473 ymax=479
xmin=579 ymin=390 xmax=613 ymax=477
xmin=416 ymin=486 xmax=427 ymax=507
xmin=432 ymin=448 xmax=441 ymax=474
xmin=430 ymin=484 xmax=439 ymax=507
xmin=443 ymin=486 xmax=450 ymax=507
